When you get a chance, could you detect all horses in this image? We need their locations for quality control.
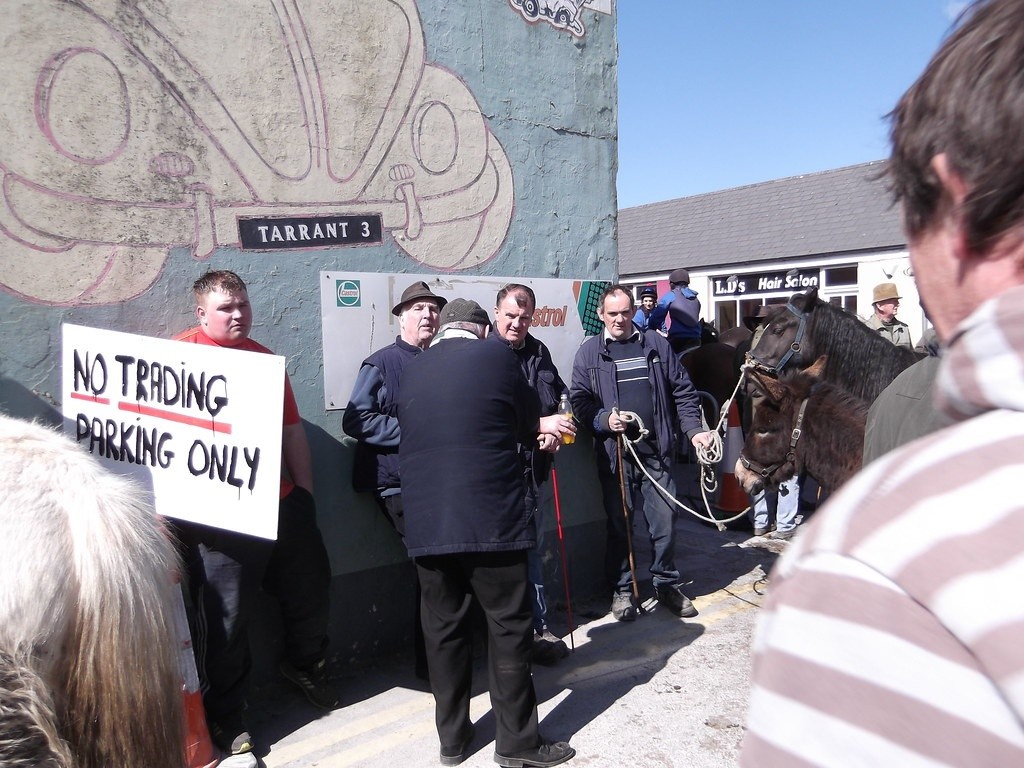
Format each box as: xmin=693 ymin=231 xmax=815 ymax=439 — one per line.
xmin=0 ymin=410 xmax=191 ymax=768
xmin=751 ymin=288 xmax=929 ymax=406
xmin=733 ymin=355 xmax=869 ymax=497
xmin=677 ymin=316 xmax=769 ymax=437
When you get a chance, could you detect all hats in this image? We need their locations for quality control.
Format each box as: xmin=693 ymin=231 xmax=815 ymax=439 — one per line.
xmin=870 ymin=284 xmax=904 ymax=304
xmin=440 ymin=298 xmax=496 ymax=333
xmin=392 ymin=282 xmax=449 ymax=316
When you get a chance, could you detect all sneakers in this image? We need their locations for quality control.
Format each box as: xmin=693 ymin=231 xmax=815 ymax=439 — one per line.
xmin=209 ymin=707 xmax=254 ymax=754
xmin=288 ymin=657 xmax=339 ymax=709
xmin=611 ymin=591 xmax=635 ymax=620
xmin=655 ymin=584 xmax=694 ymax=617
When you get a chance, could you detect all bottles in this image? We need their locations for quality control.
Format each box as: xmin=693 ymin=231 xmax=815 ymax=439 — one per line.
xmin=558 ymin=394 xmax=575 ymax=445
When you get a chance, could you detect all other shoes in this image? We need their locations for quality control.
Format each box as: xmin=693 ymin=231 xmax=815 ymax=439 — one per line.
xmin=775 ymin=523 xmax=799 ymax=539
xmin=542 ymin=629 xmax=568 ymax=660
xmin=531 ymin=630 xmax=553 ymax=664
xmin=753 ymin=520 xmax=777 ymax=536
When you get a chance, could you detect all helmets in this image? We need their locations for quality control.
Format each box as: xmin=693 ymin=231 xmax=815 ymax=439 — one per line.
xmin=668 ymin=269 xmax=691 ymax=284
xmin=639 ymin=287 xmax=658 ymax=300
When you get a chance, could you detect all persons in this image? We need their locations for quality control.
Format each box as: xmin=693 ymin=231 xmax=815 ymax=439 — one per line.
xmin=394 ymin=298 xmax=576 ymax=768
xmin=478 ymin=283 xmax=577 ymax=663
xmin=648 ymin=269 xmax=701 ymax=352
xmin=750 ymin=474 xmax=799 ymax=540
xmin=915 ymin=327 xmax=939 ymax=357
xmin=864 ymin=283 xmax=914 ymax=351
xmin=569 ymin=283 xmax=714 ymax=622
xmin=633 ymin=284 xmax=667 ymax=336
xmin=863 ymin=351 xmax=961 ymax=463
xmin=738 ymin=0 xmax=1024 ymax=766
xmin=170 ymin=271 xmax=341 ymax=754
xmin=342 ymin=279 xmax=490 ymax=683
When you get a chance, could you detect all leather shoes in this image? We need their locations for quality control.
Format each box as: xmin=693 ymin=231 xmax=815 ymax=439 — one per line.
xmin=496 ymin=734 xmax=574 ymax=767
xmin=442 ymin=717 xmax=480 ymax=763
